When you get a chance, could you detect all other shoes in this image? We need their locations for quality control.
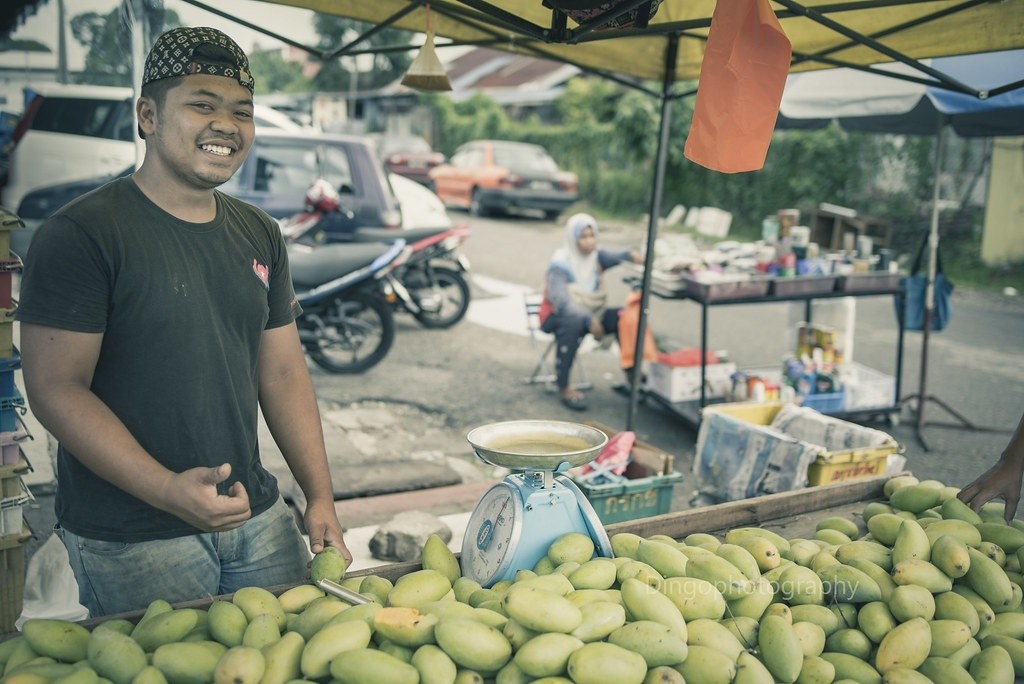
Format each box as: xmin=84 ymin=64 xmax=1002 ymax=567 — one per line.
xmin=562 ymin=396 xmax=587 ymax=410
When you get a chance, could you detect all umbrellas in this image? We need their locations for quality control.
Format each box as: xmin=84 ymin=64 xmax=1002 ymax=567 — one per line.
xmin=775 ymin=49 xmax=1024 ymax=451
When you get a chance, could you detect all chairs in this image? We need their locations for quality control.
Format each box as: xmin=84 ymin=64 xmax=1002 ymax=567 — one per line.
xmin=519 ymin=282 xmax=596 ymax=395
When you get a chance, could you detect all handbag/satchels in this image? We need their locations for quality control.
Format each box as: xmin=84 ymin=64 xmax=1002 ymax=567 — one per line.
xmin=894 ymin=231 xmax=955 ymax=331
xmin=403 ymin=32 xmax=453 ymax=91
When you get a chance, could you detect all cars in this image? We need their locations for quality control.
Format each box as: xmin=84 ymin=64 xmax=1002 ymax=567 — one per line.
xmin=0 ymin=79 xmax=453 ymax=243
xmin=363 ymin=132 xmax=448 ymax=191
xmin=426 ymin=138 xmax=584 ymax=224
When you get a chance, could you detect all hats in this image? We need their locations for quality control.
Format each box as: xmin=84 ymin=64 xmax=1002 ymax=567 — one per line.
xmin=137 ymin=27 xmax=255 ymax=140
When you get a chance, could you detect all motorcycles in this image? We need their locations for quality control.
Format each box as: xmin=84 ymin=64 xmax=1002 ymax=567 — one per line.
xmin=270 ymin=233 xmax=424 ymax=374
xmin=269 ymin=142 xmax=473 ymax=332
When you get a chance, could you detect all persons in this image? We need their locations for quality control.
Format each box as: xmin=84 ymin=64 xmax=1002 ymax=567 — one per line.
xmin=956 ymin=415 xmax=1024 ymax=527
xmin=11 ymin=24 xmax=355 ymax=618
xmin=538 ymin=213 xmax=647 ymax=412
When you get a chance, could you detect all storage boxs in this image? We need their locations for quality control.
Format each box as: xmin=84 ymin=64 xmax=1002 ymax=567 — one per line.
xmin=564 ymin=461 xmax=684 ymax=525
xmin=687 ymin=400 xmax=906 ymax=510
xmin=834 ymin=362 xmax=897 ymax=412
xmin=683 ymin=269 xmax=907 ymax=299
xmin=0 ymin=209 xmax=42 ymax=639
xmin=647 ymin=347 xmax=738 ymax=405
xmin=796 ymin=381 xmax=846 ymax=411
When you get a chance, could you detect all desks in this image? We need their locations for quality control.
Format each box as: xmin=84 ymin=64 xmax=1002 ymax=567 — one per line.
xmin=620 ymin=273 xmax=908 ymax=430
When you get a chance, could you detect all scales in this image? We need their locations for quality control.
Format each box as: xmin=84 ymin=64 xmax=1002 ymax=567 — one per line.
xmin=458 ymin=418 xmax=616 ymax=589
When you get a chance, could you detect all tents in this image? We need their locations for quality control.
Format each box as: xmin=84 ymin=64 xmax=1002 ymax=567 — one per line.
xmin=184 ymin=0 xmax=1024 ymax=433
